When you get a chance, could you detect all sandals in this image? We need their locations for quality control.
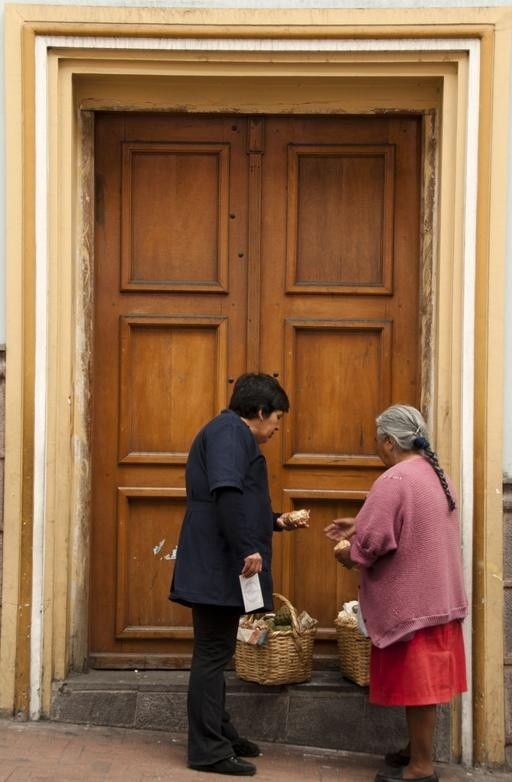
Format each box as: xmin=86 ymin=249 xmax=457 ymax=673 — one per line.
xmin=375 ymin=749 xmax=439 ymax=782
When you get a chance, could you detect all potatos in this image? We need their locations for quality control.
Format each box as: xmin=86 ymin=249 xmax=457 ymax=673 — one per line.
xmin=284 ymin=510 xmax=310 ymax=528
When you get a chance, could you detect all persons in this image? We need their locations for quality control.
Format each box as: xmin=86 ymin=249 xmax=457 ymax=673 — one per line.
xmin=322 ymin=404 xmax=468 ymax=782
xmin=169 ymin=373 xmax=310 ymax=776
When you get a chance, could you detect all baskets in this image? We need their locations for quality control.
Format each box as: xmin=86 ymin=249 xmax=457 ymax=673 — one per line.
xmin=235 ymin=592 xmax=317 ymax=686
xmin=335 ymin=618 xmax=370 ymax=687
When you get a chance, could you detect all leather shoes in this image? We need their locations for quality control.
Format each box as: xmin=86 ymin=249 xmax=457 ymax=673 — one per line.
xmin=187 ymin=738 xmax=259 ymax=775
